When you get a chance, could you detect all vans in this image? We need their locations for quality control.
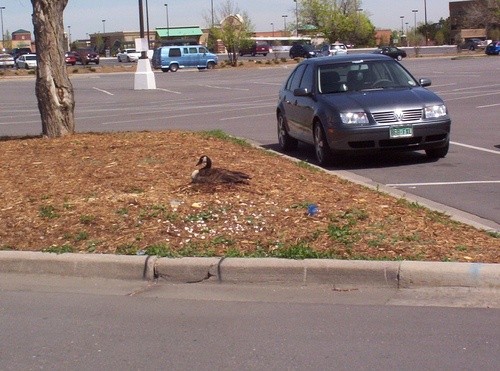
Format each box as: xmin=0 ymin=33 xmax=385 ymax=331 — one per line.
xmin=154 ymin=45 xmax=218 ymax=72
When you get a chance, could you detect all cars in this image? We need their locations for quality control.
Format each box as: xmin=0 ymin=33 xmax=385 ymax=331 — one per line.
xmin=0 ymin=48 xmax=37 ymax=69
xmin=289 ymin=44 xmax=322 ymax=59
xmin=64 ymin=49 xmax=99 ymax=66
xmin=276 ymin=53 xmax=451 ymax=168
xmin=328 ymin=43 xmax=348 ymax=56
xmin=457 ymin=39 xmax=500 ymax=56
xmin=373 ymin=46 xmax=407 ymax=61
xmin=117 ymin=49 xmax=142 ymax=63
xmin=239 ymin=41 xmax=269 ymax=57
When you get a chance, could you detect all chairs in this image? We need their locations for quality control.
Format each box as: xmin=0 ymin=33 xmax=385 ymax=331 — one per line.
xmin=345 ymin=70 xmax=379 ymax=91
xmin=320 ymin=71 xmax=341 ymax=93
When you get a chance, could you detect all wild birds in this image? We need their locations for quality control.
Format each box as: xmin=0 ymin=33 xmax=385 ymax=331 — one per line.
xmin=191 ymin=155 xmax=252 ymax=186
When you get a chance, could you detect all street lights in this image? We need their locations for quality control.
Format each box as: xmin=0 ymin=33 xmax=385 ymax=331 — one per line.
xmin=271 ymin=23 xmax=274 ymax=37
xmin=294 ymin=0 xmax=298 ymax=37
xmin=282 ymin=15 xmax=288 ymax=37
xmin=357 ymin=9 xmax=363 ymax=13
xmin=0 ymin=7 xmax=5 ymax=48
xmin=68 ymin=26 xmax=70 ymax=40
xmin=412 ymin=10 xmax=418 ymax=35
xmin=400 ymin=16 xmax=404 ymax=35
xmin=102 ymin=20 xmax=105 ymax=33
xmin=164 ymin=4 xmax=169 ymax=36
